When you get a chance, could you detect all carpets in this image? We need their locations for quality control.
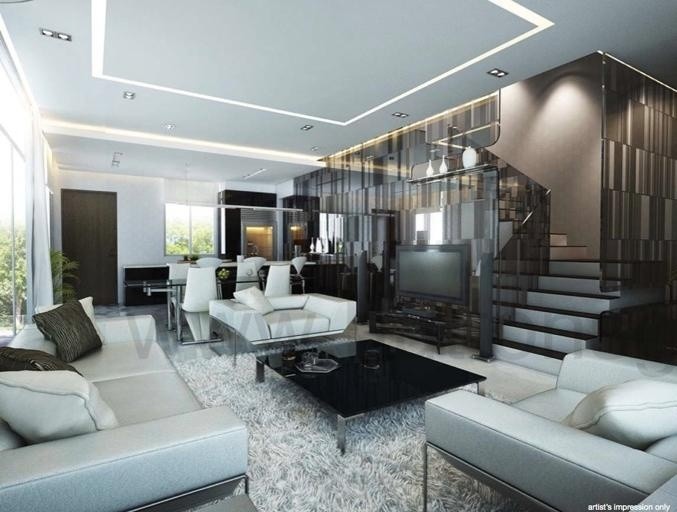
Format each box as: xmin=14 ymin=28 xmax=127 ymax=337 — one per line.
xmin=175 ymin=348 xmax=499 ymax=511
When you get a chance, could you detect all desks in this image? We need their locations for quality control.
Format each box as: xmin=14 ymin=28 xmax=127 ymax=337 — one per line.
xmin=122 ymin=278 xmax=220 ymax=341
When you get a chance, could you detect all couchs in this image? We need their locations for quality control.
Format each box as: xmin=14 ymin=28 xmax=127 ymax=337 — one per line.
xmin=0 ymin=313 xmax=250 ymax=512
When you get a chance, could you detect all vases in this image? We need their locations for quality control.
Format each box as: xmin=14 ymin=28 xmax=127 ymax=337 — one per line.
xmin=461 ymin=145 xmax=475 ymax=168
xmin=425 ymin=154 xmax=447 ymax=176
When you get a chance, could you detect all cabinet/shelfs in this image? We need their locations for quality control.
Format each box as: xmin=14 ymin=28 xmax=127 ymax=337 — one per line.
xmin=368 ymin=306 xmax=472 ymax=354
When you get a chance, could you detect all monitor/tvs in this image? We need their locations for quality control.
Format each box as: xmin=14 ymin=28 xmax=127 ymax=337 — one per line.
xmin=395 ymin=244 xmax=471 ymax=322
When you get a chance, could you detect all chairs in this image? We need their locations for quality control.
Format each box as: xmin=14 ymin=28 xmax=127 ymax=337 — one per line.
xmin=207 ymin=292 xmax=358 ymax=369
xmin=422 ymin=347 xmax=677 ymax=510
xmin=166 ymin=256 xmax=307 ymax=346
xmin=232 ymin=285 xmax=273 ymax=316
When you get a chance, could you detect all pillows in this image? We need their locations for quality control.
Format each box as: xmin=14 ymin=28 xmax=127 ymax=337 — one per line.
xmin=0 ymin=345 xmax=118 ymax=445
xmin=558 ymin=375 xmax=677 ymax=449
xmin=31 ymin=296 xmax=104 ymax=362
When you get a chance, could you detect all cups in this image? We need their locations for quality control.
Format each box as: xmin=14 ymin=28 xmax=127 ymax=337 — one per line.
xmin=281 ymin=341 xmax=296 ymax=361
xmin=365 ymin=350 xmax=382 ymax=371
